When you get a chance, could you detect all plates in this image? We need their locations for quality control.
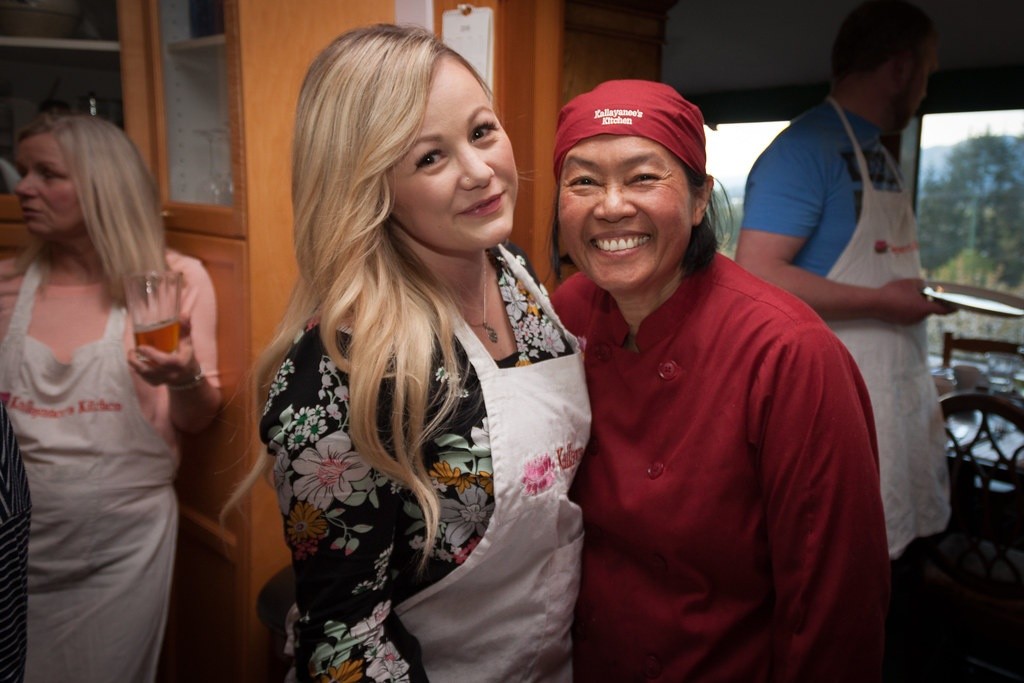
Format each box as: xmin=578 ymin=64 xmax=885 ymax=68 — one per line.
xmin=923 ymin=282 xmax=1024 ymax=318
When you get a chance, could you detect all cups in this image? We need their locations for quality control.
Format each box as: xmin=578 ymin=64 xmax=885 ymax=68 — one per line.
xmin=122 ymin=271 xmax=183 ymax=355
xmin=985 ymin=351 xmax=1016 ymax=385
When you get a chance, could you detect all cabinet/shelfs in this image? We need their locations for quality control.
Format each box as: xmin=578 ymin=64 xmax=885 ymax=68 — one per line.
xmin=0 ymin=0 xmax=397 ymax=683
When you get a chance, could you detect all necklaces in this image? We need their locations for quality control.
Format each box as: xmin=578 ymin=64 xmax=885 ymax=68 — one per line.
xmin=467 ymin=252 xmax=499 ymax=343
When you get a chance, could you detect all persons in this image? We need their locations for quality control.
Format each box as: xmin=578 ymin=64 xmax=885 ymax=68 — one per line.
xmin=550 ymin=81 xmax=890 ymax=683
xmin=734 ymin=0 xmax=955 ymax=560
xmin=223 ymin=23 xmax=591 ymax=683
xmin=0 ymin=399 xmax=31 ymax=683
xmin=0 ymin=114 xmax=221 ymax=683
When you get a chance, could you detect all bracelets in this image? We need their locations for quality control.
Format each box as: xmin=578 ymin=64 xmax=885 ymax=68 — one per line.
xmin=170 ymin=365 xmax=206 ymax=391
